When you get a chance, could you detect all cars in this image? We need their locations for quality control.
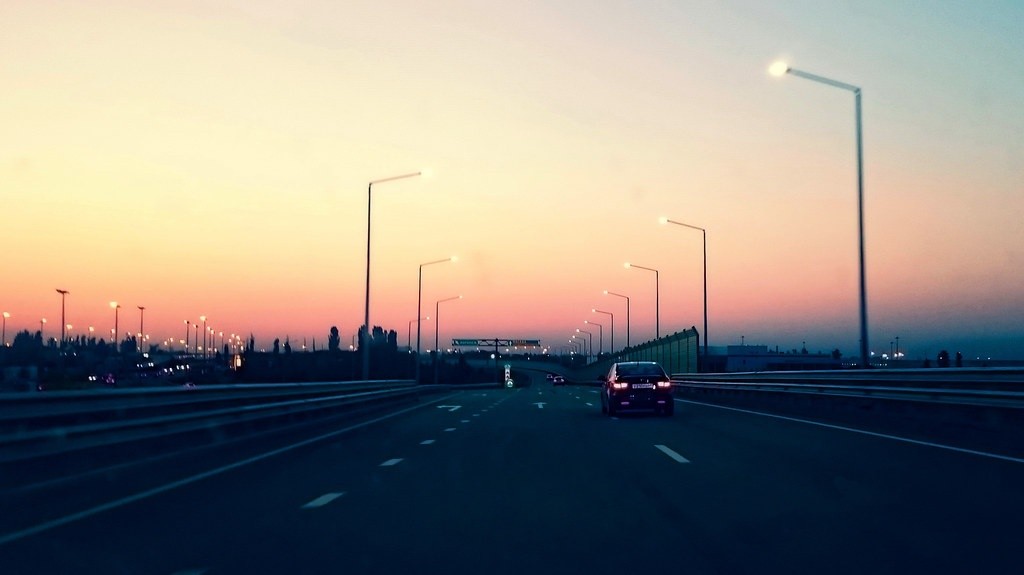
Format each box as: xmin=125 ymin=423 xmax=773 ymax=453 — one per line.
xmin=546 ymin=373 xmax=565 ymax=386
xmin=596 ymin=361 xmax=674 ymax=417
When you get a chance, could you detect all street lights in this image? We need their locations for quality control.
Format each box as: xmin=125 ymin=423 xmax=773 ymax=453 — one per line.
xmin=604 ymin=291 xmax=630 ymax=345
xmin=363 ymin=169 xmax=433 ymax=379
xmin=770 ymin=56 xmax=870 ymax=368
xmin=660 ymin=218 xmax=710 ymax=371
xmin=435 ymin=296 xmax=462 ymax=384
xmin=415 ymin=255 xmax=458 ymax=385
xmin=625 ymin=261 xmax=659 ymax=339
xmin=0 ymin=286 xmax=243 ymax=388
xmin=408 ymin=316 xmax=430 ymax=352
xmin=548 ymin=308 xmax=614 ymax=356
